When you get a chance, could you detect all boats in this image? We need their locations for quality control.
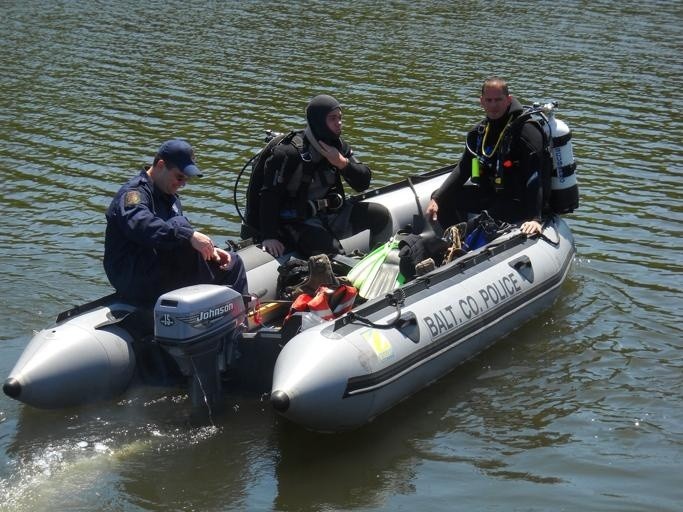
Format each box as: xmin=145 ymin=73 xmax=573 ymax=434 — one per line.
xmin=3 ymin=158 xmax=576 ymax=435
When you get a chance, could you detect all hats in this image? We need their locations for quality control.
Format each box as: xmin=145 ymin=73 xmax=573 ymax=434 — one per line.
xmin=157 ymin=138 xmax=204 ymax=179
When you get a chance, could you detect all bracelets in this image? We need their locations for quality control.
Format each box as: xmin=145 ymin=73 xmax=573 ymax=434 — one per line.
xmin=344 ymin=157 xmax=349 ymax=167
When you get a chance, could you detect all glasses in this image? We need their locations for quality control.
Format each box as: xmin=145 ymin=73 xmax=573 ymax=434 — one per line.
xmin=165 ymin=162 xmax=189 ymax=181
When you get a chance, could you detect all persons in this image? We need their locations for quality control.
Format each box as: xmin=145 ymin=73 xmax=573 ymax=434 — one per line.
xmin=99 ymin=138 xmax=251 ymax=325
xmin=420 ymin=77 xmax=555 ymax=239
xmin=244 ymin=92 xmax=394 ymax=275
